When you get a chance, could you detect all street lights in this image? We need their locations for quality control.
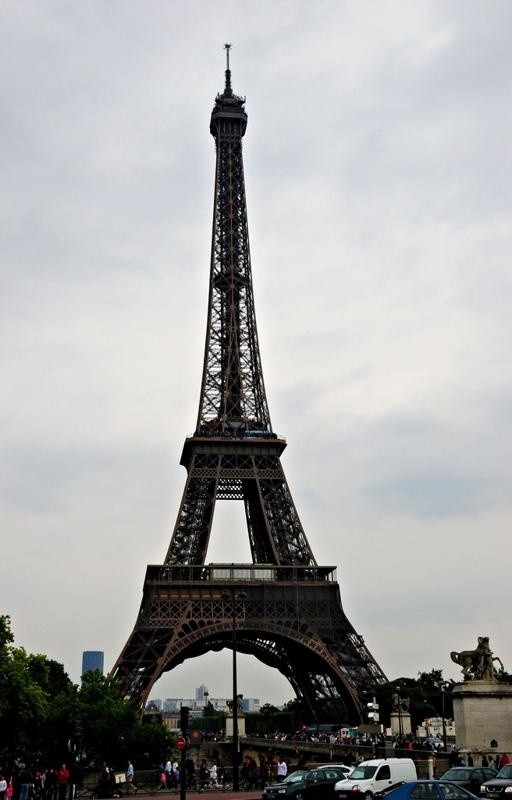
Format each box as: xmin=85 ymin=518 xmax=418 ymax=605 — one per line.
xmin=390 ymin=682 xmax=404 ymax=736
xmin=432 ymin=678 xmax=450 ymax=754
xmin=359 ymin=690 xmax=378 ymax=746
xmin=220 ymin=587 xmax=249 ymax=791
xmin=203 ymin=690 xmax=210 ymax=741
xmin=263 ymin=702 xmax=272 ymax=738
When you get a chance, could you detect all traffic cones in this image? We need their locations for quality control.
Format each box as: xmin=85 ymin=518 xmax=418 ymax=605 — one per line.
xmin=407 ymin=743 xmax=414 ymax=751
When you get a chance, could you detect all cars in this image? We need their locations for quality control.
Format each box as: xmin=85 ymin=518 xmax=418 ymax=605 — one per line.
xmin=252 ymin=727 xmax=444 ymax=752
xmin=439 ymin=767 xmax=500 ymax=796
xmin=372 ymin=780 xmax=494 ymax=800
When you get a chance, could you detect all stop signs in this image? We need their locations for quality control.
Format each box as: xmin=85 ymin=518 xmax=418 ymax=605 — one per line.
xmin=176 ymin=737 xmax=185 ymax=750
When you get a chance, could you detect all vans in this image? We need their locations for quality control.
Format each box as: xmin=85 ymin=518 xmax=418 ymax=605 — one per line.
xmin=334 ymin=756 xmax=417 ymax=800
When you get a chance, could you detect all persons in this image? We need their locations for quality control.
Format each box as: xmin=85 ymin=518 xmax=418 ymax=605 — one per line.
xmin=125 ymin=759 xmax=139 ymax=796
xmin=0 ymin=761 xmax=71 ymax=799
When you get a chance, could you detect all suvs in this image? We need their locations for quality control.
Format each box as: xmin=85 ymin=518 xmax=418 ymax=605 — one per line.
xmin=264 ymin=768 xmax=346 ymax=800
xmin=289 ymin=763 xmax=355 ymax=782
xmin=480 ymin=763 xmax=512 ymax=799
